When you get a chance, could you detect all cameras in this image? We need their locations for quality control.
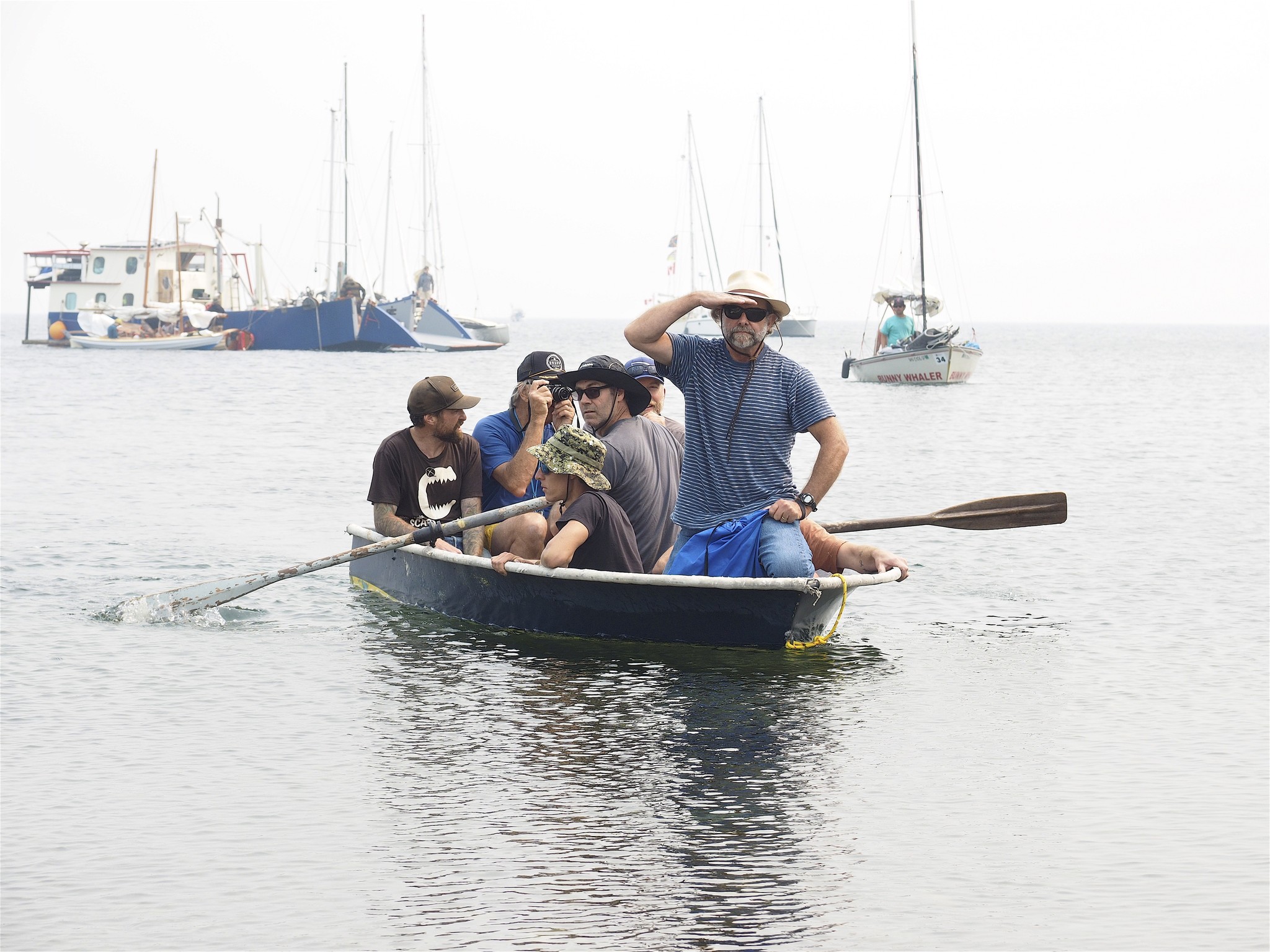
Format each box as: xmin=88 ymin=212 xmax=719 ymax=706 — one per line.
xmin=539 ymin=384 xmax=570 ymax=403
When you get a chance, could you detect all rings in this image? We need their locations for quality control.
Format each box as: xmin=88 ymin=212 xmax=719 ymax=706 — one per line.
xmin=781 ymin=516 xmax=787 ymax=520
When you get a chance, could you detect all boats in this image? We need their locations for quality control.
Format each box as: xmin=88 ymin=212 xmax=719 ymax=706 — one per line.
xmin=346 ymin=523 xmax=901 ymax=651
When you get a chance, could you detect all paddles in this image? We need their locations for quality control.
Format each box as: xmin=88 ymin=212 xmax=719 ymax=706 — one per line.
xmin=827 ymin=489 xmax=1073 ymax=535
xmin=88 ymin=490 xmax=557 ymax=627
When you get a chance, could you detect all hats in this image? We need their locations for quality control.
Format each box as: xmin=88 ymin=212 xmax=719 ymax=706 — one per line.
xmin=624 ymin=357 xmax=664 ymax=384
xmin=893 ymin=297 xmax=904 ymax=306
xmin=526 ymin=424 xmax=613 ymax=490
xmin=722 ymin=270 xmax=790 ymax=317
xmin=557 ymin=356 xmax=652 ymax=416
xmin=517 ymin=351 xmax=566 ymax=380
xmin=407 ymin=375 xmax=481 ymax=417
xmin=212 ymin=297 xmax=221 ymax=303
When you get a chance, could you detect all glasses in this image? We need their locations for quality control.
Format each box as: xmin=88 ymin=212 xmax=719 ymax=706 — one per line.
xmin=724 ymin=304 xmax=770 ymax=321
xmin=627 ymin=364 xmax=661 ymax=376
xmin=894 ymin=306 xmax=902 ymax=308
xmin=540 ymin=462 xmax=550 ymax=475
xmin=569 ymin=385 xmax=611 ymax=401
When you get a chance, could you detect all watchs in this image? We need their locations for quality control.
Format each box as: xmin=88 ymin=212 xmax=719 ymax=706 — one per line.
xmin=798 ymin=493 xmax=818 ymax=512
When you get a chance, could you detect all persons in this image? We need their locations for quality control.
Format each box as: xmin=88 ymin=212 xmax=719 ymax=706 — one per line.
xmin=339 ymin=277 xmax=366 ymax=327
xmin=880 ymin=298 xmax=915 ymax=349
xmin=367 ymin=352 xmax=909 ymax=583
xmin=415 ymin=266 xmax=435 ymax=308
xmin=206 ymin=297 xmax=226 ymax=326
xmin=624 ymin=271 xmax=849 ymax=580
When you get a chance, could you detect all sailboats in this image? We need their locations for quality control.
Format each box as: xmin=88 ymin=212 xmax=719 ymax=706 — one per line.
xmin=638 ymin=94 xmax=819 ymax=342
xmin=19 ymin=14 xmax=512 ymax=353
xmin=840 ymin=0 xmax=986 ymax=387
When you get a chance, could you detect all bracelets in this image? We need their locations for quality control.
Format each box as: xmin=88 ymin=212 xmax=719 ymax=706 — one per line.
xmin=792 ymin=498 xmax=806 ymax=520
